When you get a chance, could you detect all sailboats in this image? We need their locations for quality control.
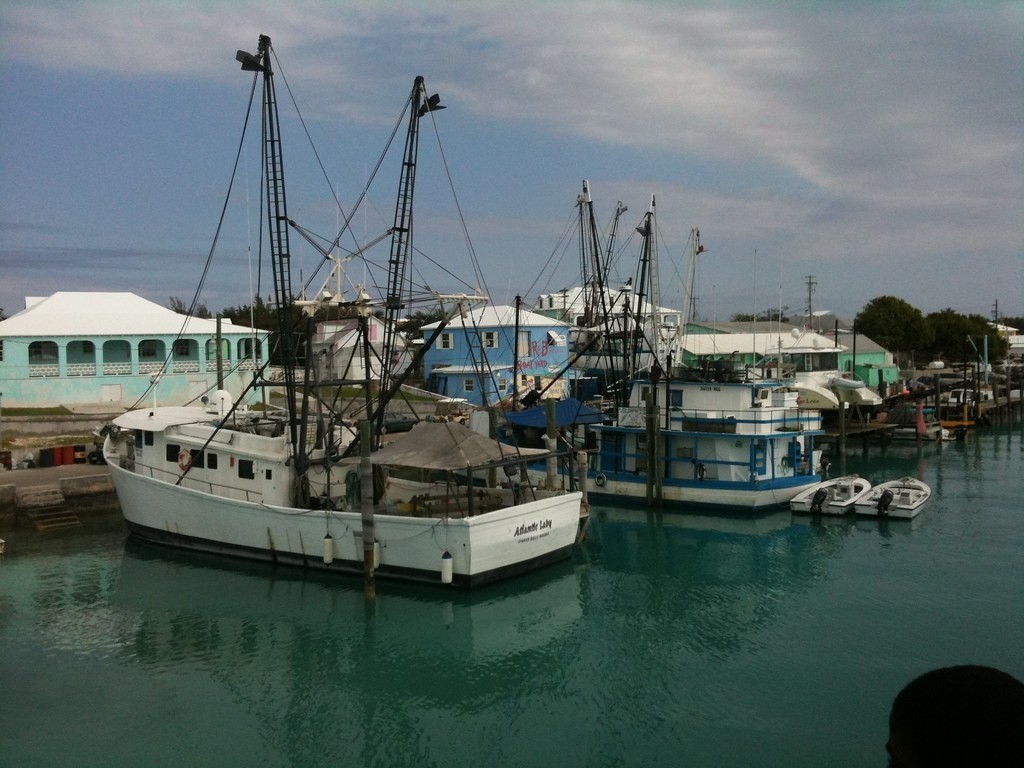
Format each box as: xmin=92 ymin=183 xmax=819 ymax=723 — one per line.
xmin=455 ymin=176 xmax=830 ymax=515
xmin=98 ymin=28 xmax=583 ymax=593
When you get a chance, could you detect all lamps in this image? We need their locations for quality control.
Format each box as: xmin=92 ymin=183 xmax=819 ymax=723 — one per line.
xmin=358 ymin=294 xmax=371 ymax=303
xmin=318 ymin=290 xmax=333 ymax=302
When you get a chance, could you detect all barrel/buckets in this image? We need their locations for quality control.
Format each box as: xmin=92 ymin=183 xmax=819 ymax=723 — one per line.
xmin=39 ymin=445 xmax=86 ymax=467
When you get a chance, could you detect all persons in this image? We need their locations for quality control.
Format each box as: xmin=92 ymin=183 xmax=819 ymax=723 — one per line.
xmin=908 ymin=378 xmax=913 ymax=391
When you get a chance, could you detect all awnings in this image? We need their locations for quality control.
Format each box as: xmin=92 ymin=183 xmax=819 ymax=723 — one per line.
xmin=548 ymin=331 xmax=562 ymax=342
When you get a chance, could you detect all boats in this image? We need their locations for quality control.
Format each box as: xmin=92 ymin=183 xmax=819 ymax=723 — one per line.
xmin=853 ymin=474 xmax=933 ymax=520
xmin=886 ymin=406 xmax=970 ymax=444
xmin=788 ymin=473 xmax=872 ymax=517
xmin=565 ymin=271 xmax=884 ymax=412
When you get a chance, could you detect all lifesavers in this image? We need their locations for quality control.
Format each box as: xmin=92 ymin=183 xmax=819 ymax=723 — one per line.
xmin=697 ymin=463 xmax=706 ymax=480
xmin=178 ymin=449 xmax=191 ymax=471
xmin=595 ymin=473 xmax=607 ymax=487
